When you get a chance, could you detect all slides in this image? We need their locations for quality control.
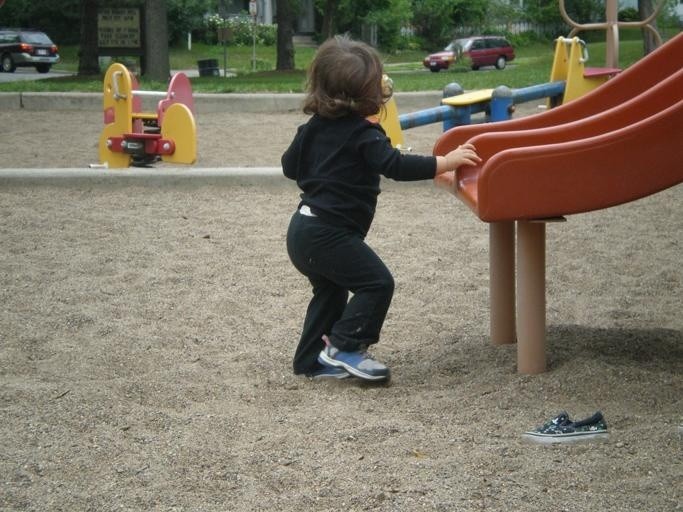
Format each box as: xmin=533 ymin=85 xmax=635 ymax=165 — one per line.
xmin=432 ymin=28 xmax=682 ymax=220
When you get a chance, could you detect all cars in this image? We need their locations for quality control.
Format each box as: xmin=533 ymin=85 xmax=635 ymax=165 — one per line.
xmin=0 ymin=28 xmax=61 ymax=73
xmin=424 ymin=36 xmax=514 ymax=73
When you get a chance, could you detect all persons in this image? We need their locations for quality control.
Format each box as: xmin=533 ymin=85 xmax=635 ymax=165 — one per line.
xmin=278 ymin=31 xmax=483 ymax=382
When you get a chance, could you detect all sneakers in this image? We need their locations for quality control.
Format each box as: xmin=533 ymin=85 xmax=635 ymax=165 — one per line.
xmin=316 ymin=339 xmax=391 ymax=383
xmin=292 ymin=359 xmax=351 ymax=380
xmin=520 ymin=409 xmax=610 ymax=445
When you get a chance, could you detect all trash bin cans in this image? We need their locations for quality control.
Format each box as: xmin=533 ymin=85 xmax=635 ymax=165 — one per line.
xmin=197 ymin=59 xmax=219 ymax=75
